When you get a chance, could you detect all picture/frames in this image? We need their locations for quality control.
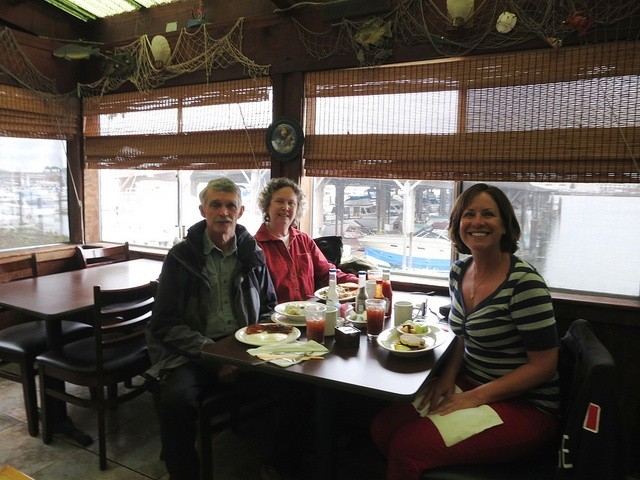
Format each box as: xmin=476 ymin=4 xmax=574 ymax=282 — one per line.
xmin=265 ymin=118 xmax=304 ymax=162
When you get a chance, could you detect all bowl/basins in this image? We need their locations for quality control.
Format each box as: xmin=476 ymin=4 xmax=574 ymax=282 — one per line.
xmin=397 ymin=324 xmax=432 ymax=339
xmin=346 ymin=313 xmax=388 ymax=330
xmin=273 ymin=300 xmax=331 ymax=321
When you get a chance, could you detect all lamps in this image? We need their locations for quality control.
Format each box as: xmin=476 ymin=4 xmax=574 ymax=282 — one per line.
xmin=150 ymin=34 xmax=171 ymax=70
xmin=445 ymin=0 xmax=474 ymax=28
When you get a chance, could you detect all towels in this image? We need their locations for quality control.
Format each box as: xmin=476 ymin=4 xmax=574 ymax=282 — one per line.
xmin=245 ymin=339 xmax=330 ymax=368
xmin=411 ymin=383 xmax=504 ymax=447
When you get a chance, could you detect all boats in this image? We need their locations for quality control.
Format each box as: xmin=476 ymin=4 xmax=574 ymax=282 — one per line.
xmin=358 ymin=217 xmax=457 ymax=269
xmin=321 ymin=194 xmax=420 ymax=235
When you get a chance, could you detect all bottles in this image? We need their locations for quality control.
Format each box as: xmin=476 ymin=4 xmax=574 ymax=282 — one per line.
xmin=353 ymin=269 xmax=369 ymax=323
xmin=380 ymin=269 xmax=392 ymax=325
xmin=325 ymin=269 xmax=340 ymax=326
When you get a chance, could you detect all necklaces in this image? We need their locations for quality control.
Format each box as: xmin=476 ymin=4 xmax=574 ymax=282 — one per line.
xmin=469 ymin=249 xmax=503 ymax=300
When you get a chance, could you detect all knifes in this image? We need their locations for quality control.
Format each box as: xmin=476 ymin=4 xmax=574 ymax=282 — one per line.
xmin=256 ymin=354 xmax=324 ymax=362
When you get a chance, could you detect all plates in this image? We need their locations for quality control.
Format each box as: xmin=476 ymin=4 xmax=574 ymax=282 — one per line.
xmin=376 ymin=322 xmax=448 ymax=358
xmin=314 ymin=282 xmax=359 ymax=301
xmin=233 ymin=323 xmax=302 ymax=347
xmin=270 ymin=312 xmax=309 ymax=327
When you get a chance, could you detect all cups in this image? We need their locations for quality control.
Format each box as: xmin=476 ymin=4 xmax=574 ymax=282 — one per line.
xmin=304 ymin=304 xmax=327 ymax=346
xmin=367 ymin=270 xmax=383 ymax=280
xmin=365 ymin=280 xmax=378 ymax=298
xmin=365 ymin=298 xmax=387 ymax=339
xmin=325 ymin=306 xmax=338 ymax=337
xmin=393 ymin=300 xmax=421 ymax=327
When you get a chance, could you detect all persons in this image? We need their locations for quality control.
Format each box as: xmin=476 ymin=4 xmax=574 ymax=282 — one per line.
xmin=252 ymin=176 xmax=359 ymax=305
xmin=142 ymin=177 xmax=306 ymax=480
xmin=369 ymin=183 xmax=563 ymax=480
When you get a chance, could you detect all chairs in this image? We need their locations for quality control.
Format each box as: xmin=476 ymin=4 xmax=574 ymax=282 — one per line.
xmin=1 ymin=252 xmax=97 ymax=437
xmin=156 ymin=275 xmax=273 ymax=479
xmin=75 ymin=241 xmax=133 ymax=389
xmin=35 ymin=279 xmax=159 ymax=471
xmin=420 ymin=317 xmax=614 ymax=479
